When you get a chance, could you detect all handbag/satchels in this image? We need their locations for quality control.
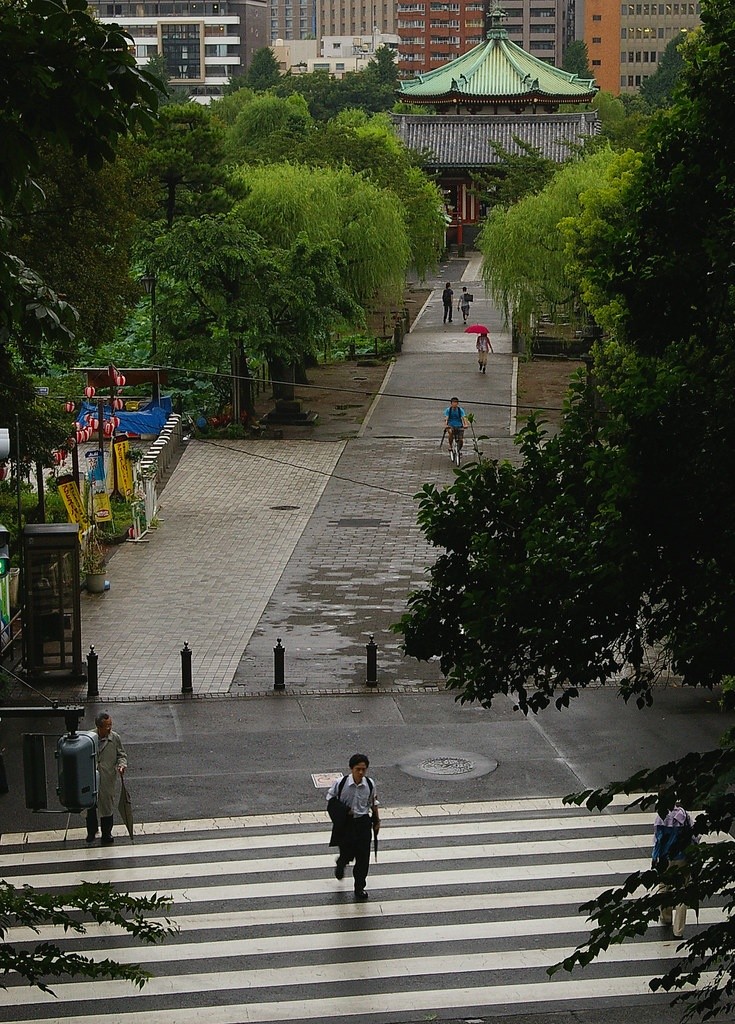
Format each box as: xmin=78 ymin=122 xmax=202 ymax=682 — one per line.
xmin=464 ymin=293 xmax=473 ymax=302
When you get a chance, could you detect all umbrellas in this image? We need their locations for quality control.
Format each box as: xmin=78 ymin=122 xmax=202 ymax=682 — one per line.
xmin=439 ymin=426 xmax=450 ymax=449
xmin=464 ymin=322 xmax=490 ymax=347
xmin=116 ymin=766 xmax=134 ymax=845
xmin=371 ymin=794 xmax=380 ymax=863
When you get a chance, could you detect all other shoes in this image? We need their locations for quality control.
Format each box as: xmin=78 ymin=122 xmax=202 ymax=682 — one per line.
xmin=86 ymin=834 xmax=95 ymax=842
xmin=102 ymin=835 xmax=114 ymax=842
xmin=355 ymin=889 xmax=368 ymax=898
xmin=448 ymin=445 xmax=452 ymax=451
xmin=335 ymin=861 xmax=344 ymax=879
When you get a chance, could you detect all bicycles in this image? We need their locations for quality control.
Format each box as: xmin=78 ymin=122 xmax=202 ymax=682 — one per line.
xmin=444 ymin=425 xmax=468 ymax=467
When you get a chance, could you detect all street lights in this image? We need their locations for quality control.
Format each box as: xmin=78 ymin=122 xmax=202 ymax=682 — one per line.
xmin=139 ymin=263 xmax=159 ymax=366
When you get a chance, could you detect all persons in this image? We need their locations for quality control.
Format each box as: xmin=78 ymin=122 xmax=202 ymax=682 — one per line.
xmin=457 ymin=286 xmax=470 ymax=325
xmin=324 ymin=752 xmax=381 ymax=898
xmin=651 ymin=804 xmax=702 ymax=939
xmin=476 ymin=332 xmax=495 ymax=374
xmin=444 ymin=397 xmax=469 ymax=455
xmin=86 ymin=712 xmax=127 ymax=843
xmin=442 ymin=282 xmax=454 ymax=324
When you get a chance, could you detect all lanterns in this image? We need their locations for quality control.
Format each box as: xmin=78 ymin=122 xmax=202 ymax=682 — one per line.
xmin=0 ymin=464 xmax=8 ymax=480
xmin=54 ymin=370 xmax=126 ymax=463
xmin=129 ymin=528 xmax=138 ymax=538
